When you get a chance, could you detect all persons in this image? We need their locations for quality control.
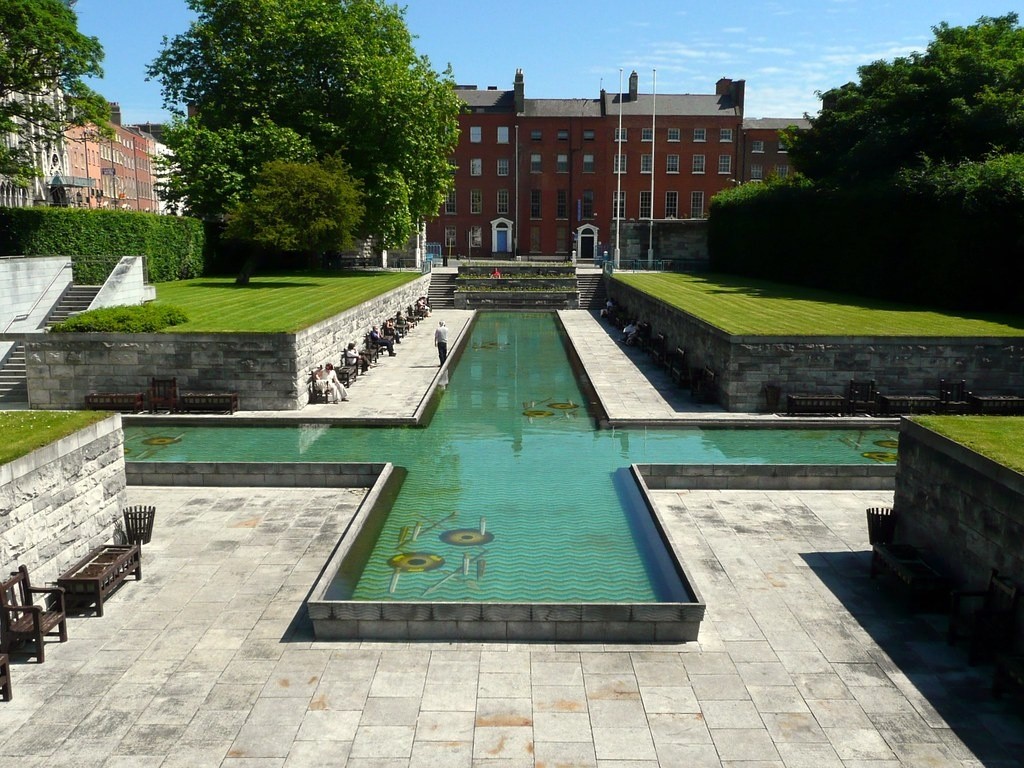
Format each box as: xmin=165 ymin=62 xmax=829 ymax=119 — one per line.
xmin=434 ymin=320 xmax=448 ymax=367
xmin=396 ymin=311 xmax=414 ymax=330
xmin=384 ymin=318 xmax=401 ymax=343
xmin=370 ymin=325 xmax=396 ymax=357
xmin=600 ymin=297 xmax=613 ymax=317
xmin=491 ymin=266 xmax=500 ymax=277
xmin=315 ymin=363 xmax=349 ymax=404
xmin=617 ymin=320 xmax=638 ymax=343
xmin=347 ymin=342 xmax=377 ymax=376
xmin=598 ymin=297 xmax=615 ymax=315
xmin=421 ymin=296 xmax=431 ymax=317
xmin=624 ymin=322 xmax=646 ymax=346
xmin=418 ymin=298 xmax=429 ymax=318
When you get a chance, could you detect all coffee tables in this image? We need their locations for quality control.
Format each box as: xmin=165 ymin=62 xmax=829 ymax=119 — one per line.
xmin=973 ymin=393 xmax=1024 ymax=416
xmin=56 ymin=543 xmax=142 ymax=618
xmin=180 ymin=390 xmax=240 ymax=416
xmin=879 ymin=393 xmax=940 ymax=415
xmin=787 ymin=393 xmax=846 ymax=418
xmin=84 ymin=391 xmax=145 ymax=414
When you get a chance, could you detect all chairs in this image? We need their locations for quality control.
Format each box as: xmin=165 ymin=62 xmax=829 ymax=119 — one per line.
xmin=939 ymin=378 xmax=974 ymax=415
xmin=0 ymin=565 xmax=69 ymax=664
xmin=308 ymin=294 xmax=434 ymax=406
xmin=602 ymin=293 xmax=718 ymax=404
xmin=866 ymin=506 xmax=1024 ymax=693
xmin=146 ymin=377 xmax=180 ymax=415
xmin=848 ymin=378 xmax=877 ymax=417
